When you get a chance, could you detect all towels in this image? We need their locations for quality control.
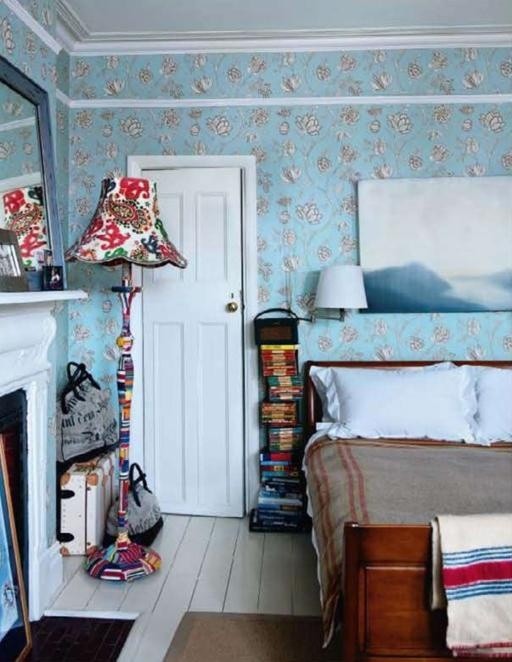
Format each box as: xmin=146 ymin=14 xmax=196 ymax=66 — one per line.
xmin=430 ymin=513 xmax=512 ymax=659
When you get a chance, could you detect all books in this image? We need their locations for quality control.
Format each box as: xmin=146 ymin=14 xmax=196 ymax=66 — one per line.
xmin=252 ymin=342 xmax=306 ymax=531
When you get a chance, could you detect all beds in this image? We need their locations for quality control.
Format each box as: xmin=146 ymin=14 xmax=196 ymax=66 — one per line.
xmin=302 ymin=360 xmax=512 ymax=661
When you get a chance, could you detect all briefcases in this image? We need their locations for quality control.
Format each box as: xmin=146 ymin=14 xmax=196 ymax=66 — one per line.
xmin=60 ymin=450 xmax=116 ymax=556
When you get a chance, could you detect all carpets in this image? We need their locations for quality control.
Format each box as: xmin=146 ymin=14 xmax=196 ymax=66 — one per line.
xmin=0 ymin=616 xmax=136 ymax=662
xmin=163 ymin=611 xmax=345 ymax=662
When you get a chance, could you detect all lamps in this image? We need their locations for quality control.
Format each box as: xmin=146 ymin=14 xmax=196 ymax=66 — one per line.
xmin=309 ymin=265 xmax=368 ymax=322
xmin=61 ymin=174 xmax=188 ymax=582
xmin=1 ymin=184 xmax=53 ymax=271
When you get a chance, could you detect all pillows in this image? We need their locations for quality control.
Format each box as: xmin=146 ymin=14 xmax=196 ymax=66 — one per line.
xmin=308 ymin=362 xmax=512 ymax=447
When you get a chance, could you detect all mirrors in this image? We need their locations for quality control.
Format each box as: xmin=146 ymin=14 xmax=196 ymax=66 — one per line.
xmin=0 ymin=53 xmax=66 ymax=291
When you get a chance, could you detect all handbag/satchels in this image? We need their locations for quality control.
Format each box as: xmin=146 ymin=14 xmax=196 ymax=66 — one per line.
xmin=57 ymin=361 xmax=119 ymax=464
xmin=103 ymin=463 xmax=163 ymax=549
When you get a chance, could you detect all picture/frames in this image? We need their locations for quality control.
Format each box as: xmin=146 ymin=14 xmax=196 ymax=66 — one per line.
xmin=0 ymin=229 xmax=29 ymax=293
xmin=0 ymin=435 xmax=34 ymax=662
xmin=42 ymin=265 xmax=63 ymax=291
